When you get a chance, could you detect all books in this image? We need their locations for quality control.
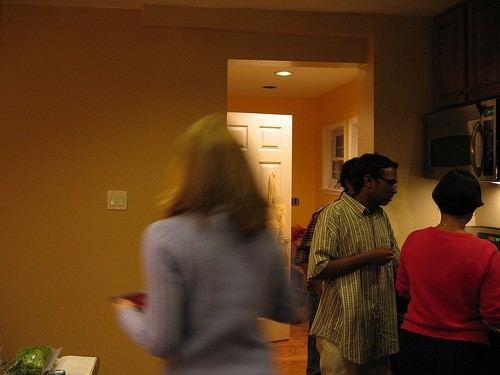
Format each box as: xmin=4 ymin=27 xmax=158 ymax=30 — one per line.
xmin=49 ymin=355 xmax=98 ymax=375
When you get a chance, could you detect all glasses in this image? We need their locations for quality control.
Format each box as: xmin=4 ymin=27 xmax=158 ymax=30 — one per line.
xmin=379 ymin=176 xmax=397 ymax=189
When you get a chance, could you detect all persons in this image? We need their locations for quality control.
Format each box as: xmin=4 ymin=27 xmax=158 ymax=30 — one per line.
xmin=393 ymin=169 xmax=500 ymax=375
xmin=292 ymin=156 xmax=361 ymax=375
xmin=109 ymin=114 xmax=312 ymax=375
xmin=306 ymin=152 xmax=401 ymax=375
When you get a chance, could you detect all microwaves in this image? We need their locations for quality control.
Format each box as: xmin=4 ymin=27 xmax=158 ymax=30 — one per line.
xmin=422 ymin=99 xmax=500 ymax=184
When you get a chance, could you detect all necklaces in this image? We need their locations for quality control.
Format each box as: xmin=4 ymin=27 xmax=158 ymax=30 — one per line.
xmin=439 ymin=224 xmax=462 ymax=229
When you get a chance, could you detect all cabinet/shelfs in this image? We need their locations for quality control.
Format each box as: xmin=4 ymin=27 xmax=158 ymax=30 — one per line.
xmin=431 ymin=0 xmax=500 ymax=111
